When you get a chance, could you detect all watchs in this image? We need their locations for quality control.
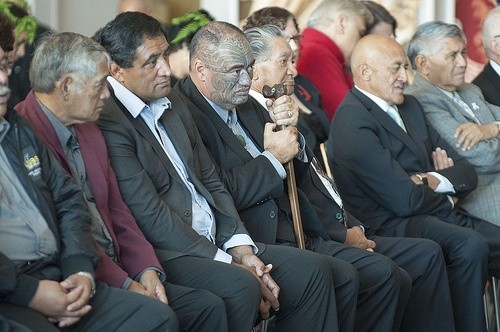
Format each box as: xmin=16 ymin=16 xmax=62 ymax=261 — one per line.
xmin=414 ymin=171 xmax=428 ymax=187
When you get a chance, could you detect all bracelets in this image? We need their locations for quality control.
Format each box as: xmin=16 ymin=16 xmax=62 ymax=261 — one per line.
xmin=496 ymin=124 xmax=500 ymax=140
xmin=78 ymin=270 xmax=96 ymax=299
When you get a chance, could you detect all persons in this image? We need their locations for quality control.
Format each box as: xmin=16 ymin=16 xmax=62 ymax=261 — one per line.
xmin=96 ymin=11 xmax=340 ymax=332
xmin=0 ymin=0 xmax=500 ymax=332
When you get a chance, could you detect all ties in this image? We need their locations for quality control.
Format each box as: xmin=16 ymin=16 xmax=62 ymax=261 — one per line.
xmin=387 ymin=105 xmax=407 ymax=134
xmin=453 ymin=94 xmax=482 ymax=125
xmin=311 ymin=158 xmax=348 ymax=228
